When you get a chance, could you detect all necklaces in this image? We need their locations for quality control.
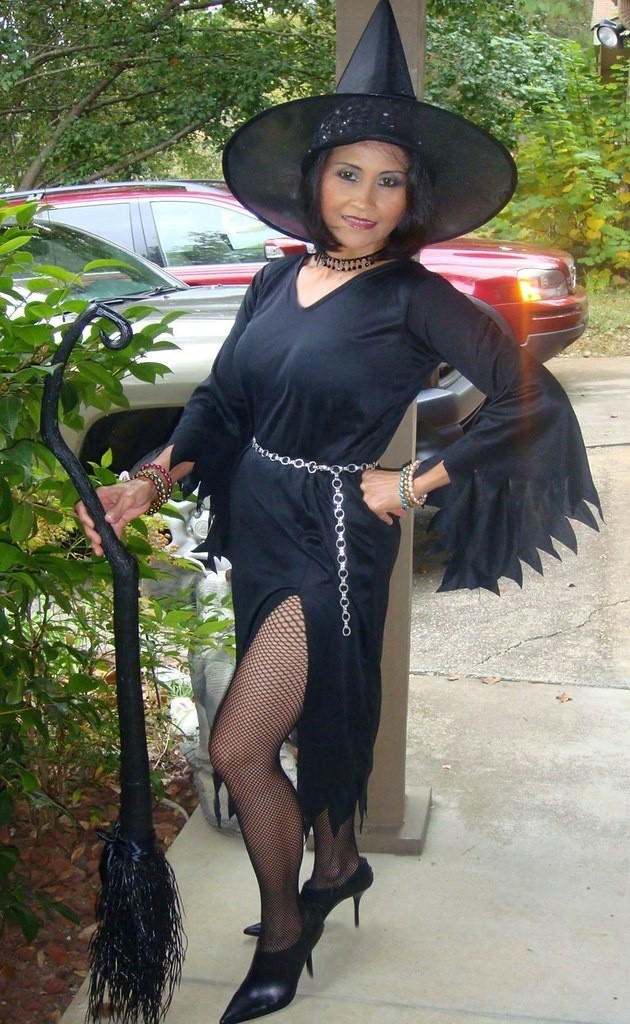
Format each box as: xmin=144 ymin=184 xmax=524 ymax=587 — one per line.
xmin=313 ymin=245 xmax=391 ymax=271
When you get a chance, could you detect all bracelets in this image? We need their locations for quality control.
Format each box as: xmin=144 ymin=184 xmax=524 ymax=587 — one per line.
xmin=396 ymin=459 xmax=429 ymax=512
xmin=131 ymin=463 xmax=173 ymax=517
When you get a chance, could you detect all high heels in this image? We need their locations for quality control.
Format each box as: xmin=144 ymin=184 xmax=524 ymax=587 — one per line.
xmin=219 ymin=906 xmax=325 ymax=1024
xmin=244 ymin=856 xmax=373 ymax=937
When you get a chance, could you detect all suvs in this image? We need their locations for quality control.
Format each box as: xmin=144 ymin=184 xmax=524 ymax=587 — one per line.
xmin=0 ymin=178 xmax=589 ymax=369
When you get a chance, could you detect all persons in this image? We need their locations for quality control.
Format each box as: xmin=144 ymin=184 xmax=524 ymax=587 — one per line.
xmin=75 ymin=9 xmax=607 ymax=1023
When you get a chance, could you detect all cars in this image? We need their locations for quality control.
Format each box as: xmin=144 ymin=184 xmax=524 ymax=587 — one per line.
xmin=0 ymin=215 xmax=496 ymax=577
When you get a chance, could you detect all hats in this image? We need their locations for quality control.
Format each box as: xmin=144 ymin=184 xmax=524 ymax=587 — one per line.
xmin=221 ymin=0 xmax=519 ymax=248
